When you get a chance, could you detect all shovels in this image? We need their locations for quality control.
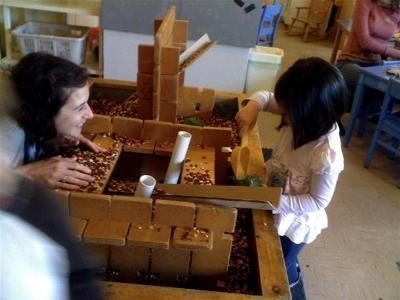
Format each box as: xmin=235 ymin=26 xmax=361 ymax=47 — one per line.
xmin=230 ymin=126 xmax=250 ymax=180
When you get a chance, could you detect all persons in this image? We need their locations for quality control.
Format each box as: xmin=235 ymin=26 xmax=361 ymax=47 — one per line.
xmin=0 ymin=53 xmax=107 ymax=189
xmin=234 ymin=56 xmax=349 ymax=300
xmin=336 ymin=0 xmax=400 ymax=136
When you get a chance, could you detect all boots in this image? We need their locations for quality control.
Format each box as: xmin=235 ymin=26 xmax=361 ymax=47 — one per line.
xmin=290 ymin=267 xmax=306 ymax=300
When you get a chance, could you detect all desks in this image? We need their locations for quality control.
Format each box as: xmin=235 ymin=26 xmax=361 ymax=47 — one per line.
xmin=343 ymin=60 xmax=399 ymax=167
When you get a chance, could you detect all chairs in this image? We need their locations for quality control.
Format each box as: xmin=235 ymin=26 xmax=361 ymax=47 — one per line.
xmin=286 ymin=0 xmax=333 ymax=42
xmin=363 ymin=75 xmax=400 ymax=189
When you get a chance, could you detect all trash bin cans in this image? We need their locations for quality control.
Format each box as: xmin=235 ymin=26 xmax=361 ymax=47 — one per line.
xmin=246 ymin=45 xmax=284 ymax=96
